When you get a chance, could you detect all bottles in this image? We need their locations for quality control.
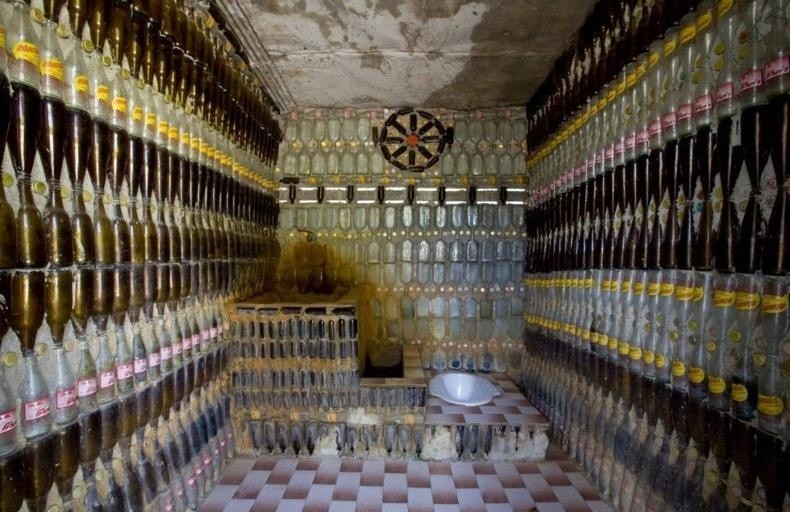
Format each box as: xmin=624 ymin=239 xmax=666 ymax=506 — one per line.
xmin=1 ymin=0 xmax=790 ymax=512
